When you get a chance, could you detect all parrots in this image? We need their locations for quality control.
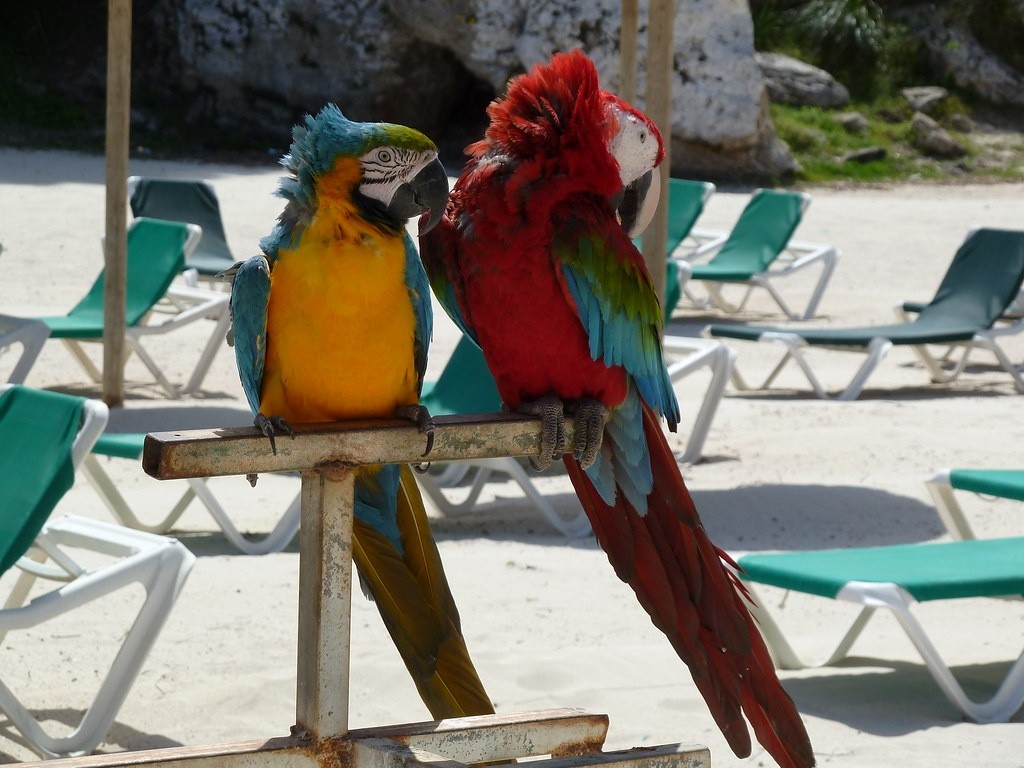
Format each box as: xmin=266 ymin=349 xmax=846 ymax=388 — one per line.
xmin=213 ymin=107 xmax=498 ymax=724
xmin=419 ymin=48 xmax=817 ymax=767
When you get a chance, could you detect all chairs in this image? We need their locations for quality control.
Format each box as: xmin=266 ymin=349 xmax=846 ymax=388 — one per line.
xmin=0 ymin=178 xmax=1024 ymax=760
